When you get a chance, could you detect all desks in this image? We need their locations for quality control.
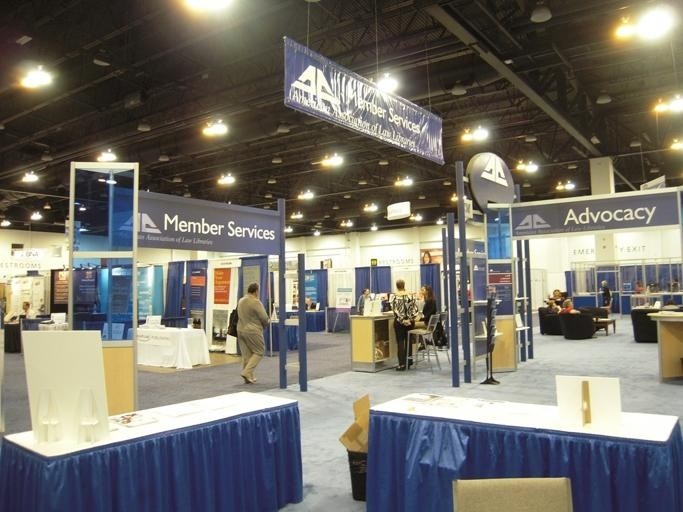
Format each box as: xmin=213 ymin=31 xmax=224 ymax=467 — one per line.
xmin=102 ymin=339 xmax=138 ymax=415
xmin=23 ymin=318 xmax=50 ymax=330
xmin=286 ymin=309 xmax=325 ymax=332
xmin=127 ymin=327 xmax=211 ymax=369
xmin=646 ymin=311 xmax=683 ymax=383
xmin=1 ymin=391 xmax=304 ymax=512
xmin=262 ymin=319 xmax=300 ymax=350
xmin=349 ymin=315 xmax=400 ymax=372
xmin=4 ymin=324 xmax=21 ymax=353
xmin=366 ymin=391 xmax=682 ymax=512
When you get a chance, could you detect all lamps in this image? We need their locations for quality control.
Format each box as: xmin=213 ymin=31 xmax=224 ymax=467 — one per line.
xmin=63 ymin=262 xmax=99 ymax=272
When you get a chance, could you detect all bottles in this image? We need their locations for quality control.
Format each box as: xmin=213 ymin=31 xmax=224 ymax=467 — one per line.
xmin=192 ymin=316 xmax=201 ymax=329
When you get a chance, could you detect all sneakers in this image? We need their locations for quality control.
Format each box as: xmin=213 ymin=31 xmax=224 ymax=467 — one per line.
xmin=396 ymin=359 xmax=414 ymax=371
xmin=239 ymin=371 xmax=258 ymax=385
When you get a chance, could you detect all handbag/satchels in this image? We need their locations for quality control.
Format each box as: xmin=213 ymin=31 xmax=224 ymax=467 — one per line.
xmin=226 ymin=305 xmax=238 ymax=338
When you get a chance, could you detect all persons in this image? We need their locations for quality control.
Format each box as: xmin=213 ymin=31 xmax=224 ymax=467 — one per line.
xmin=391 ymin=279 xmax=419 ymax=371
xmin=305 ymin=296 xmax=320 ymax=312
xmin=635 ymin=281 xmax=646 ymax=294
xmin=546 ymin=289 xmax=581 ymax=315
xmin=12 ymin=301 xmax=36 ymax=323
xmin=601 ymin=280 xmax=611 ymax=318
xmin=235 ymin=282 xmax=269 ymax=384
xmin=417 ymin=285 xmax=446 ymax=352
xmin=357 ymin=288 xmax=372 ymax=315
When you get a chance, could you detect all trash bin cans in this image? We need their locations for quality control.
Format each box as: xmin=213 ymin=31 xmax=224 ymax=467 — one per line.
xmin=347 ymin=448 xmax=368 ymax=502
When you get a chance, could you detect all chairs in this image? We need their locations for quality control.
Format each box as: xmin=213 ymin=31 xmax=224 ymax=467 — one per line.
xmin=558 ymin=312 xmax=596 ymax=339
xmin=630 ymin=308 xmax=658 ymax=343
xmin=452 ymin=477 xmax=573 ymax=512
xmin=579 ymin=306 xmax=608 ymax=318
xmin=406 ymin=310 xmax=451 ymax=373
xmin=539 ymin=307 xmax=564 ymax=335
